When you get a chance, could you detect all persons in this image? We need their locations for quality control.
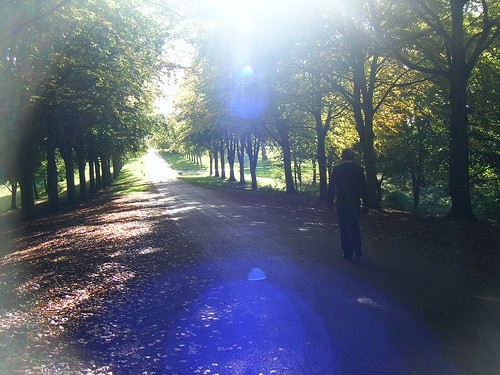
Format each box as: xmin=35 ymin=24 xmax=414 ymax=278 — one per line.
xmin=327 ymin=148 xmax=369 ymax=260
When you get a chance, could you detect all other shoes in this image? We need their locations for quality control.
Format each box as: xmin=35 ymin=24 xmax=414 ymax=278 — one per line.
xmin=355 ymin=250 xmax=362 ymax=257
xmin=342 ymin=255 xmax=352 ymax=261
